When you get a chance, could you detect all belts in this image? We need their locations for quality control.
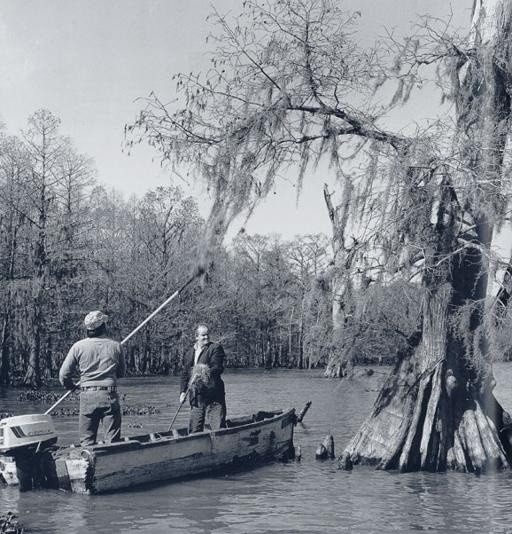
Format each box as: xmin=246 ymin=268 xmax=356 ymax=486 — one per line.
xmin=81 ymin=386 xmax=114 ymax=391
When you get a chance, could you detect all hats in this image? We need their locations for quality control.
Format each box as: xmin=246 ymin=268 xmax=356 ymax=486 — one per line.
xmin=84 ymin=310 xmax=108 ymax=330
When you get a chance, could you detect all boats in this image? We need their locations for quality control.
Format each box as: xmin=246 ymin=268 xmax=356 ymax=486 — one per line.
xmin=0 ymin=399 xmax=312 ymax=494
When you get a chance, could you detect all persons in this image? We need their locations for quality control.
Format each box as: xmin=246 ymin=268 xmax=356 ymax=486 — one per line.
xmin=180 ymin=322 xmax=228 ymax=435
xmin=57 ymin=310 xmax=127 ymax=448
